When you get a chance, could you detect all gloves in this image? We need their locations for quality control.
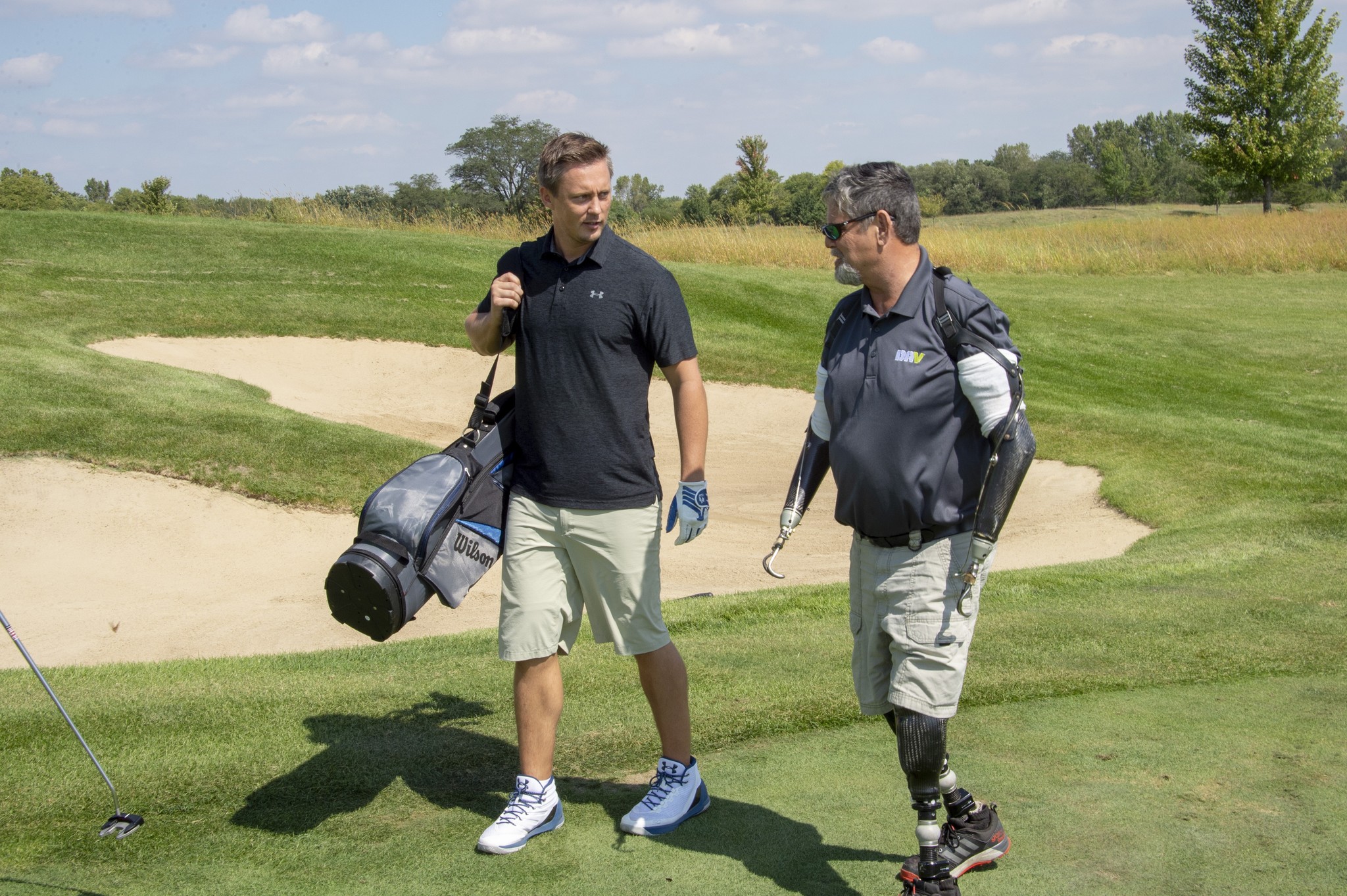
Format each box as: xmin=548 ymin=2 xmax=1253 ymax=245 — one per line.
xmin=665 ymin=481 xmax=709 ymax=546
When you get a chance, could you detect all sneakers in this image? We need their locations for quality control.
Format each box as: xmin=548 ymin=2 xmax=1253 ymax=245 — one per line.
xmin=620 ymin=755 xmax=710 ymax=836
xmin=898 ymin=877 xmax=962 ymax=896
xmin=900 ymin=804 xmax=1011 ymax=882
xmin=477 ymin=774 xmax=564 ymax=854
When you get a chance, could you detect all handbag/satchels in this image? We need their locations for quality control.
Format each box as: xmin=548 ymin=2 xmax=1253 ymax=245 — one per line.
xmin=324 ymin=384 xmax=515 ymax=643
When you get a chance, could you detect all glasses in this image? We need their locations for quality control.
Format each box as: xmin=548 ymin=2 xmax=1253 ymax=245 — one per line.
xmin=822 ymin=211 xmax=894 ymax=242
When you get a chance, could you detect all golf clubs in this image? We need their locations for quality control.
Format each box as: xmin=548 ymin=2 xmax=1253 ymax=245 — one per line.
xmin=0 ymin=609 xmax=145 ymax=842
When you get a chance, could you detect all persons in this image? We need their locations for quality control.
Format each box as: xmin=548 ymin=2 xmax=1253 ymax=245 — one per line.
xmin=765 ymin=161 xmax=1035 ymax=896
xmin=465 ymin=134 xmax=710 ymax=856
